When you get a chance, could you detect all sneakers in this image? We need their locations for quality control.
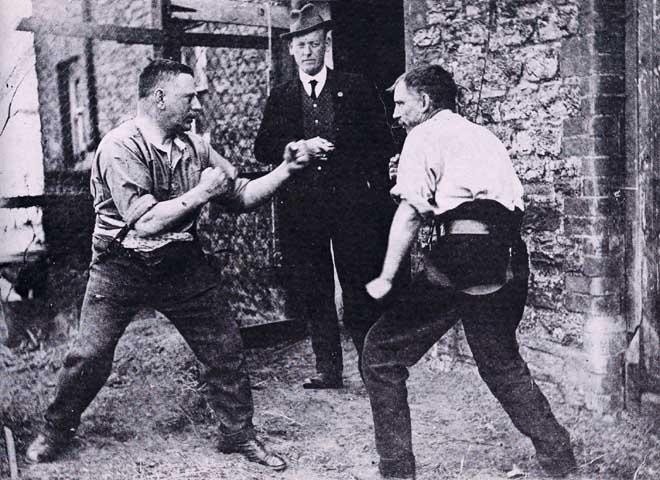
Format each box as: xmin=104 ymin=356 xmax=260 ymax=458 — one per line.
xmin=528 ymin=446 xmax=579 ymax=477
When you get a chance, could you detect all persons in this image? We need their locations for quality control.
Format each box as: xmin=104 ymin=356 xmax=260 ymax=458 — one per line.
xmin=251 ymin=1 xmax=396 ymax=392
xmin=356 ymin=63 xmax=582 ymax=479
xmin=19 ymin=54 xmax=312 ymax=470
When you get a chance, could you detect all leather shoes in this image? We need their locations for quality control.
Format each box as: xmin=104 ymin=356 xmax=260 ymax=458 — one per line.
xmin=217 ymin=435 xmax=286 ymax=472
xmin=24 ymin=430 xmax=66 ymax=466
xmin=302 ymin=371 xmax=344 ymax=389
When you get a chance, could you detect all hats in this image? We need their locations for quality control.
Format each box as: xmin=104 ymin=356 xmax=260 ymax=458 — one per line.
xmin=280 ymin=2 xmax=337 ymax=40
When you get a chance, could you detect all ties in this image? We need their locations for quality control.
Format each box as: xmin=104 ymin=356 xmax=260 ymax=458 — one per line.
xmin=308 ymin=79 xmax=318 ymax=100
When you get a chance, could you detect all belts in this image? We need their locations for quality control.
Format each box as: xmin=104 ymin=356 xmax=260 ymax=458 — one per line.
xmin=440 ymin=217 xmax=492 ymax=238
